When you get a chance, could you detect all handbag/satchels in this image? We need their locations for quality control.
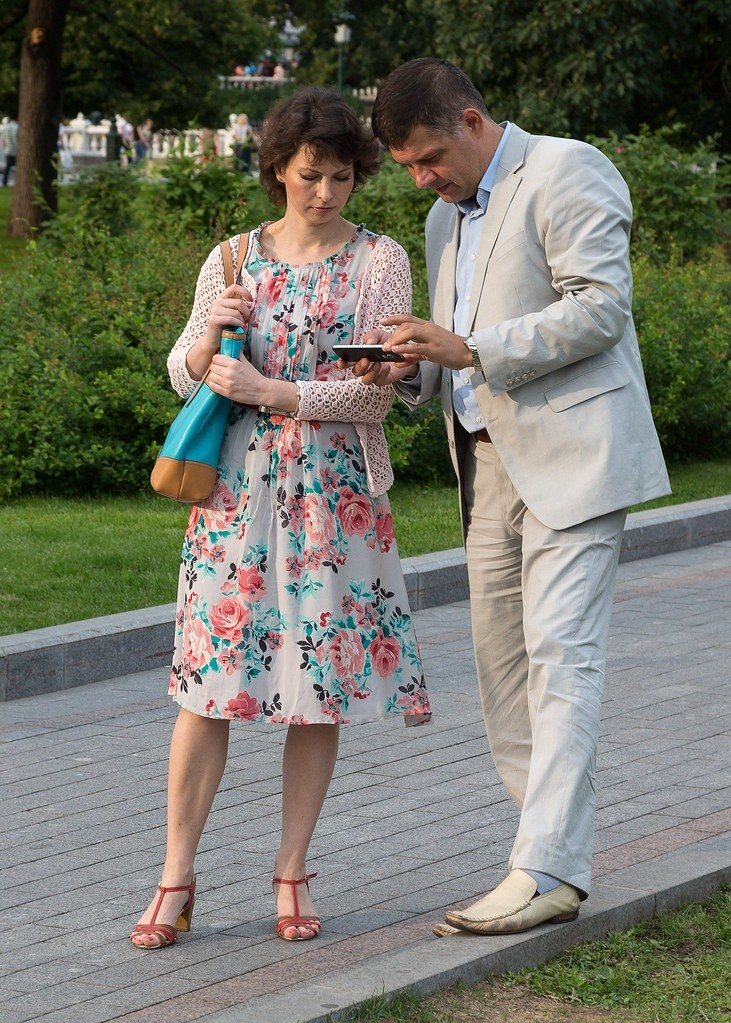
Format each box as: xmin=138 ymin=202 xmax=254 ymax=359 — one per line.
xmin=149 ymin=231 xmax=253 ymax=507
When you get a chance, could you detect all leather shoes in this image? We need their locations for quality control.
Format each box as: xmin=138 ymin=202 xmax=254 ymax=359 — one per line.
xmin=444 ymin=868 xmax=580 ymax=935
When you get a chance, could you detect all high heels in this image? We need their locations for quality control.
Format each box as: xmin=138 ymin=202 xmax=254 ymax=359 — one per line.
xmin=270 ymin=873 xmax=322 ymax=942
xmin=127 ymin=873 xmax=197 ymax=951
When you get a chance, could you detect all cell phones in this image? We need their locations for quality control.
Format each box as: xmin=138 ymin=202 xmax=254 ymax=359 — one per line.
xmin=332 ymin=343 xmax=406 ymax=362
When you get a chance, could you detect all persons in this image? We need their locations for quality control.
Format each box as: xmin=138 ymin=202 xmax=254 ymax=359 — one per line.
xmin=337 ymin=57 xmax=680 ymax=944
xmin=0 ymin=108 xmax=255 ymax=190
xmin=130 ymin=86 xmax=437 ymax=953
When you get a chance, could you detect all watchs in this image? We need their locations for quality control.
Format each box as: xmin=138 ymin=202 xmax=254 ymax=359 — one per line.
xmin=464 ymin=335 xmax=484 ymax=374
